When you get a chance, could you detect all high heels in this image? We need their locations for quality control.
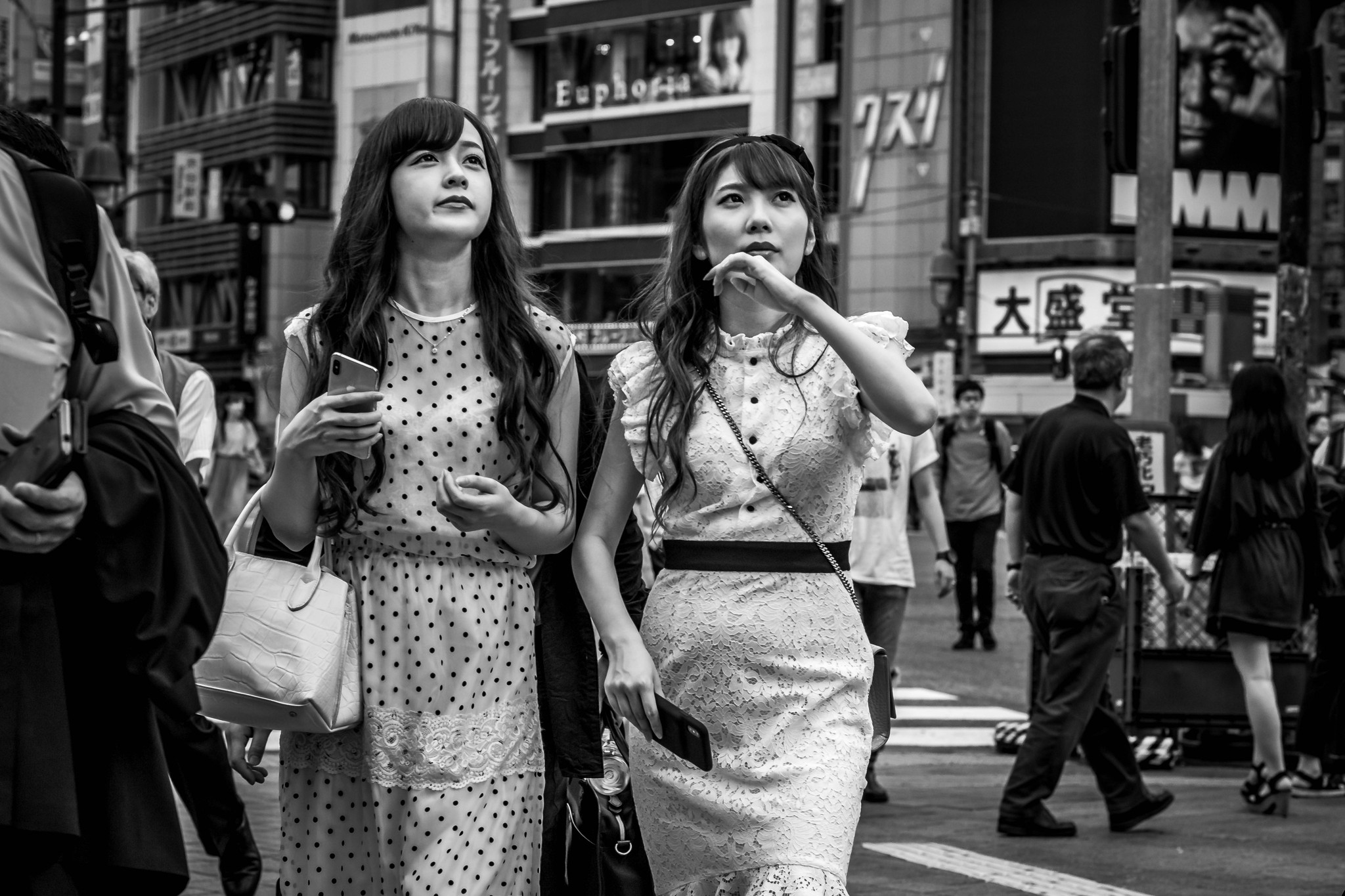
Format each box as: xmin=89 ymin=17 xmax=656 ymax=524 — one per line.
xmin=1241 ymin=762 xmax=1293 ymax=820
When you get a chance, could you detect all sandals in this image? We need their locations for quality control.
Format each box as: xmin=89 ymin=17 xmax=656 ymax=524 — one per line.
xmin=1291 ymin=770 xmax=1345 ymax=797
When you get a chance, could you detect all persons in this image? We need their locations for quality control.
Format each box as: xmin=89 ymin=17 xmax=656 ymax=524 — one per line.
xmin=0 ymin=106 xmax=258 ymax=896
xmin=1176 ymin=356 xmax=1345 ymax=822
xmin=699 ymin=5 xmax=750 ymax=96
xmin=849 ymin=378 xmax=1012 ymax=802
xmin=1176 ymin=1 xmax=1293 ymax=167
xmin=572 ymin=135 xmax=939 ymax=896
xmin=996 ymin=334 xmax=1183 ymax=838
xmin=226 ymin=99 xmax=648 ymax=896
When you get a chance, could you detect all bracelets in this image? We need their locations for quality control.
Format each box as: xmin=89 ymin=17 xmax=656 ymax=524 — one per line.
xmin=1182 ymin=567 xmax=1203 ymax=584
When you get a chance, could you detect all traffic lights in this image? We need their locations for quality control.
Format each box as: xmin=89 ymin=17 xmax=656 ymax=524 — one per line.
xmin=1051 ymin=346 xmax=1069 ymax=380
xmin=223 ymin=190 xmax=300 ymax=224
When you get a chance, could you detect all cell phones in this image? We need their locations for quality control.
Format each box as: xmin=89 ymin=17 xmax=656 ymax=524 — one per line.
xmin=648 ymin=692 xmax=713 ymax=770
xmin=327 ymin=352 xmax=380 ymax=459
xmin=1 ymin=402 xmax=70 ymax=497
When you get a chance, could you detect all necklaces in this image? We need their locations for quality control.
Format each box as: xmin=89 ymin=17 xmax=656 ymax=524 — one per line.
xmin=387 ymin=296 xmax=484 ymax=358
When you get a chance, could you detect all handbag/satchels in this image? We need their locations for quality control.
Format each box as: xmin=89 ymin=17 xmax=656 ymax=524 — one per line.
xmin=191 ymin=483 xmax=366 ymax=735
xmin=564 ymin=707 xmax=653 ymax=896
xmin=870 ymin=641 xmax=896 ymax=752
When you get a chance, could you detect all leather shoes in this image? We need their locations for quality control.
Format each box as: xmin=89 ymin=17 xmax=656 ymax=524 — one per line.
xmin=219 ymin=807 xmax=262 ymax=896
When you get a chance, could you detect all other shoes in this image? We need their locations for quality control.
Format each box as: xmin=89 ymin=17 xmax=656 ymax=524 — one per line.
xmin=996 ymin=812 xmax=1077 ymax=837
xmin=974 ymin=619 xmax=996 ymax=651
xmin=953 ymin=635 xmax=974 ymax=651
xmin=861 ymin=753 xmax=889 ymax=804
xmin=1108 ymin=791 xmax=1174 ymax=832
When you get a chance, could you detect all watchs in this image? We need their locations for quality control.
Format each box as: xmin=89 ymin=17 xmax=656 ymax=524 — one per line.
xmin=1003 ymin=558 xmax=1022 ymax=574
xmin=933 ymin=547 xmax=962 ymax=566
